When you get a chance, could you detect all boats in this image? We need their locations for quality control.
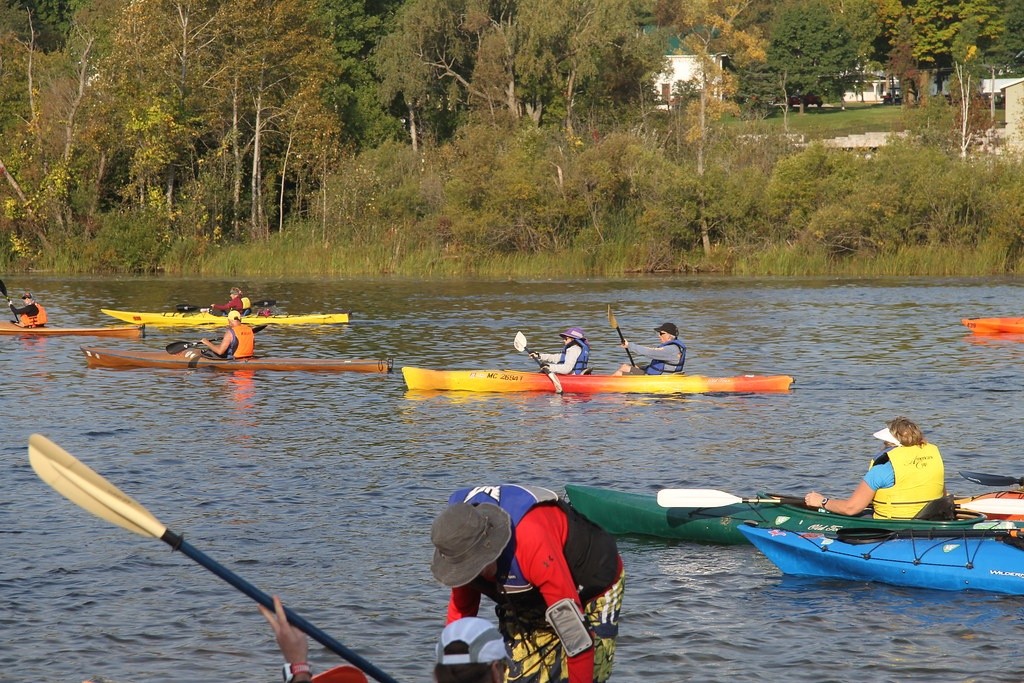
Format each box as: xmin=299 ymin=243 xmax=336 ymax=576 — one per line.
xmin=561 ymin=482 xmax=1023 ymax=545
xmin=737 ymin=521 xmax=1023 ymax=600
xmin=0 ymin=319 xmax=145 ymax=339
xmin=400 ymin=366 xmax=797 ymax=395
xmin=101 ymin=308 xmax=352 ymax=325
xmin=960 ymin=317 xmax=1024 ymax=335
xmin=79 ymin=344 xmax=396 ymax=374
xmin=949 ymin=489 xmax=1024 ymax=523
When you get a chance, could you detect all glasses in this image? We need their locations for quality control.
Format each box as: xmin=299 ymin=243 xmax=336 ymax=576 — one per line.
xmin=660 ymin=332 xmax=665 ymax=335
xmin=23 ymin=297 xmax=31 ymax=300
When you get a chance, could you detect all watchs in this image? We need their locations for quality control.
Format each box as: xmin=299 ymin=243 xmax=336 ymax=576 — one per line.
xmin=281 ymin=661 xmax=312 ymax=683
xmin=821 ymin=498 xmax=830 ymax=510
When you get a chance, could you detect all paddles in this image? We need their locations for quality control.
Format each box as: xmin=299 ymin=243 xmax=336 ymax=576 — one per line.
xmin=27 ymin=434 xmax=399 ymax=683
xmin=513 ymin=331 xmax=562 ymax=393
xmin=607 ymin=303 xmax=635 ymax=367
xmin=165 ymin=324 xmax=268 ymax=355
xmin=177 ymin=299 xmax=277 ymax=314
xmin=657 ymin=489 xmax=806 ymax=508
xmin=827 ymin=527 xmax=1023 ymax=539
xmin=958 ymin=469 xmax=1024 ymax=486
xmin=0 ymin=279 xmax=19 ymax=322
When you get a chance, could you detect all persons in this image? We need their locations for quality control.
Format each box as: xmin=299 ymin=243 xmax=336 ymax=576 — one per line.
xmin=431 ymin=483 xmax=625 ymax=683
xmin=8 ymin=292 xmax=47 ymax=328
xmin=805 ymin=416 xmax=947 ymax=521
xmin=611 ymin=322 xmax=687 ymax=376
xmin=200 ymin=310 xmax=255 ymax=359
xmin=211 ymin=287 xmax=252 ymax=317
xmin=529 ymin=327 xmax=590 ymax=376
xmin=257 ymin=594 xmax=515 ymax=683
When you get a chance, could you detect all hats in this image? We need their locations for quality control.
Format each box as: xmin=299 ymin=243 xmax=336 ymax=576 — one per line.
xmin=228 ymin=311 xmax=241 ymax=320
xmin=431 ymin=501 xmax=512 ymax=588
xmin=22 ymin=293 xmax=34 ymax=300
xmin=230 ymin=287 xmax=239 ymax=295
xmin=873 ymin=428 xmax=901 ymax=447
xmin=653 ymin=323 xmax=679 ymax=337
xmin=435 ymin=617 xmax=511 ymax=665
xmin=559 ymin=327 xmax=584 ymax=341
